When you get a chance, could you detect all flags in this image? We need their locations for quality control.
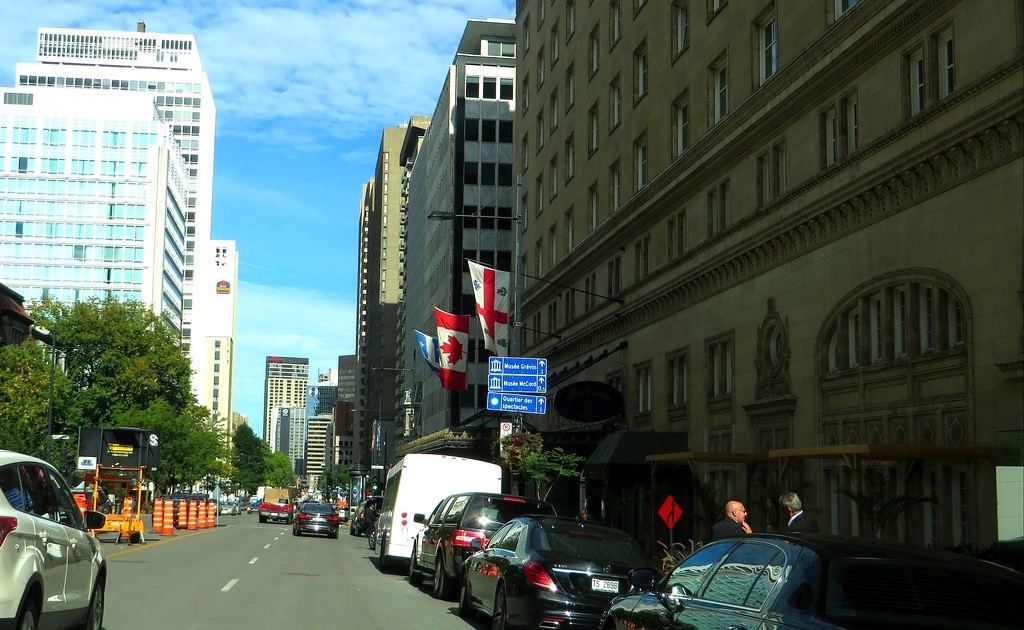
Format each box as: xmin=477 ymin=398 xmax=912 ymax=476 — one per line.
xmin=415 ymin=330 xmax=440 ymax=378
xmin=434 ymin=306 xmax=470 ymax=391
xmin=468 ymin=260 xmax=509 ymax=357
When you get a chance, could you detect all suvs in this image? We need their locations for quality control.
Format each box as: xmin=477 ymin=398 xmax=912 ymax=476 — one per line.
xmin=350 ymin=495 xmax=384 ymax=537
xmin=409 ymin=492 xmax=560 ymax=600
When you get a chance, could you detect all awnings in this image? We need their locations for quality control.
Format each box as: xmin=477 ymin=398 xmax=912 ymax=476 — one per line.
xmin=536 ymin=430 xmax=688 ymax=465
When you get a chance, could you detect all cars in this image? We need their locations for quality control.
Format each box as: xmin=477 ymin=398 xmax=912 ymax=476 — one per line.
xmin=152 ymin=493 xmax=263 ymax=528
xmin=294 ymin=486 xmax=359 ymax=523
xmin=599 ymin=527 xmax=1024 ymax=630
xmin=458 ymin=513 xmax=662 ymax=630
xmin=0 ymin=449 xmax=108 ymax=630
xmin=292 ymin=503 xmax=340 ymax=538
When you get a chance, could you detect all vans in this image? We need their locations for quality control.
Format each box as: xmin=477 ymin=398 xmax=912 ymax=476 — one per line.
xmin=374 ymin=453 xmax=503 ymax=572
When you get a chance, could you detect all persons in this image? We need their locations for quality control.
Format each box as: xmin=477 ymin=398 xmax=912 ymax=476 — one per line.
xmin=579 ymin=509 xmax=589 ymax=520
xmin=108 ymin=492 xmax=115 ymax=506
xmin=779 ymin=493 xmax=819 ymax=532
xmin=713 ymin=501 xmax=752 ymax=540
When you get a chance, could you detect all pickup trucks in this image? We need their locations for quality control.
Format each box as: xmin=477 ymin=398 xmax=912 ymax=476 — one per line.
xmin=258 ymin=495 xmax=295 ymax=525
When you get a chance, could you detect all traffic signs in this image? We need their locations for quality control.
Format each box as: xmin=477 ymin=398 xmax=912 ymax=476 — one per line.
xmin=488 ymin=375 xmax=547 ymax=392
xmin=488 ymin=356 xmax=548 ymax=376
xmin=486 ymin=392 xmax=546 ymax=415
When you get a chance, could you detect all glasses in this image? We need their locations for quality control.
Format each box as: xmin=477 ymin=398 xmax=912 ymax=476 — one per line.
xmin=782 ymin=507 xmax=788 ymax=512
xmin=734 ymin=510 xmax=748 ymax=513
